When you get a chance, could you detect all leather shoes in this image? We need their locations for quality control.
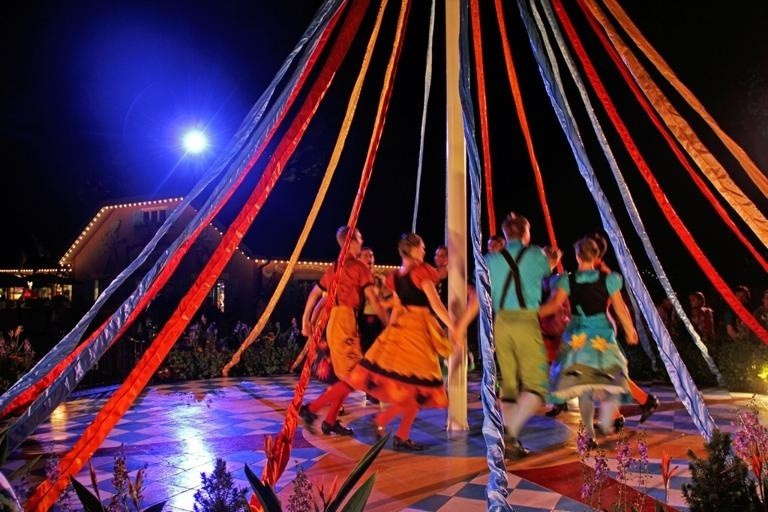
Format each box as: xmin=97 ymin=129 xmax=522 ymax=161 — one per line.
xmin=545 ymin=403 xmax=569 ymax=418
xmin=320 ymin=418 xmax=353 ymax=437
xmin=298 ymin=403 xmax=317 ymax=434
xmin=504 ymin=439 xmax=530 ymax=460
xmin=391 ymin=433 xmax=430 ymax=451
xmin=586 ymin=393 xmax=660 ymax=451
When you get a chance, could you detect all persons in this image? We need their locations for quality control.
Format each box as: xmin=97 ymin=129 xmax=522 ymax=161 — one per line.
xmin=283 ymin=206 xmax=663 ymax=457
xmin=193 ymin=313 xmax=212 ymax=349
xmin=752 ymin=289 xmax=768 ymax=332
xmin=721 ymin=285 xmax=751 ymax=341
xmin=686 ymin=290 xmax=717 ymax=341
xmin=282 ymin=317 xmax=299 ymax=340
xmin=652 ymin=294 xmax=678 ymax=331
